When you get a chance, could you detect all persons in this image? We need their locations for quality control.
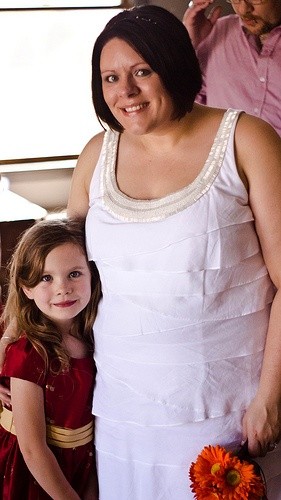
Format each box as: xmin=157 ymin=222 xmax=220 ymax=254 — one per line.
xmin=0 ymin=5 xmax=281 ymax=499
xmin=183 ymin=0 xmax=281 ymax=139
xmin=0 ymin=219 xmax=100 ymax=499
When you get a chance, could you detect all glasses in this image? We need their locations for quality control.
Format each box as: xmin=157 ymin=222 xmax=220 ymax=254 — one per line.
xmin=227 ymin=0 xmax=270 ymax=4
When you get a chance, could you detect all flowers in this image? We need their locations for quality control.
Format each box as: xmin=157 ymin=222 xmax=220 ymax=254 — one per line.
xmin=189 ymin=444 xmax=267 ymax=500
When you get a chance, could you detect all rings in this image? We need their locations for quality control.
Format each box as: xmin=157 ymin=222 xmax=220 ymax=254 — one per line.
xmin=270 ymin=443 xmax=279 ymax=448
